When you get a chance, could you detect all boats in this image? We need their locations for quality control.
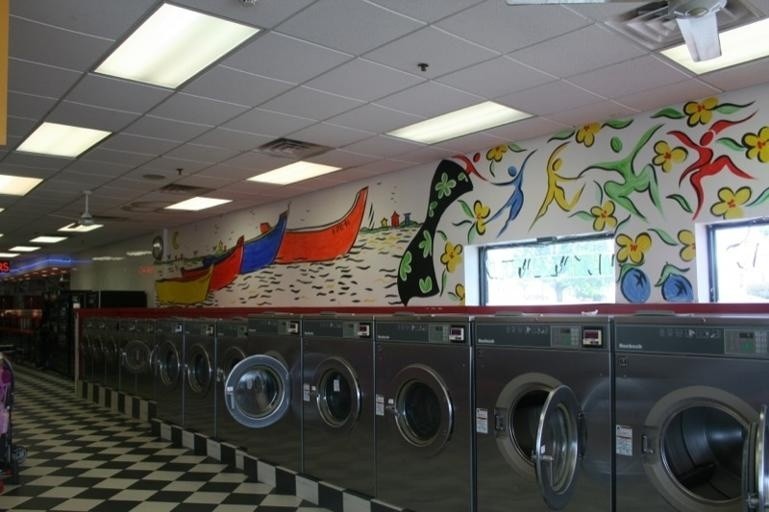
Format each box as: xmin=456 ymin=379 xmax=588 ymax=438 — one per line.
xmin=180 ymin=235 xmax=244 ymax=292
xmin=260 ymin=186 xmax=368 ymax=265
xmin=201 ymin=209 xmax=287 ymax=276
xmin=155 ymin=263 xmax=214 ymax=305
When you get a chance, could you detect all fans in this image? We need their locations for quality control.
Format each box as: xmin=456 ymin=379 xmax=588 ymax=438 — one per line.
xmin=502 ymin=0 xmax=730 ymax=64
xmin=47 ymin=189 xmax=130 ymax=226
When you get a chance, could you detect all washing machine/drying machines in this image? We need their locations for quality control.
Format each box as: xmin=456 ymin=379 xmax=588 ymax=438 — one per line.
xmin=375 ymin=312 xmax=473 ymax=512
xmin=301 ymin=308 xmax=373 ymax=500
xmin=470 ymin=312 xmax=615 ymax=512
xmin=614 ymin=311 xmax=769 ymax=510
xmin=78 ymin=310 xmax=301 ymax=475
